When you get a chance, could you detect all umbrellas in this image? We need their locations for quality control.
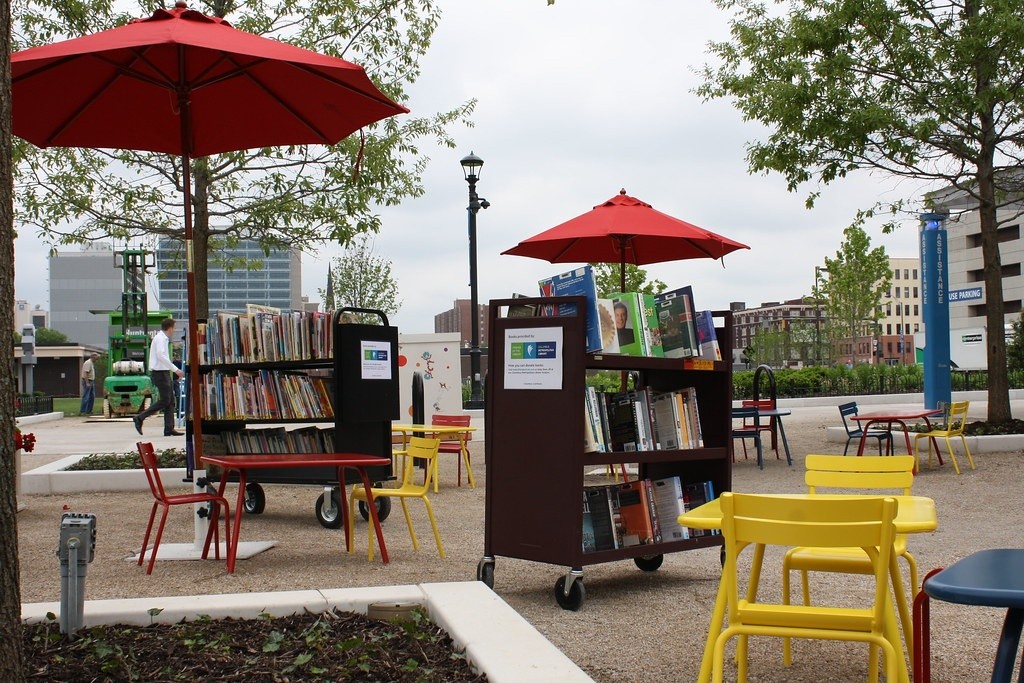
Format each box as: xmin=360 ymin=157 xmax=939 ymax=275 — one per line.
xmin=8 ymin=0 xmax=408 ymax=549
xmin=502 ymin=188 xmax=749 ymax=291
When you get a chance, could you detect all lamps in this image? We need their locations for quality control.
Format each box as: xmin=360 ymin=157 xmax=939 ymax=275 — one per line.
xmin=58 ymin=513 xmax=97 ymax=565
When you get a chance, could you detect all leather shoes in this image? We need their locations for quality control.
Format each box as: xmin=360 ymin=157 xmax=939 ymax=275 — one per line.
xmin=164 ymin=429 xmax=185 ymax=436
xmin=133 ymin=415 xmax=143 ymax=435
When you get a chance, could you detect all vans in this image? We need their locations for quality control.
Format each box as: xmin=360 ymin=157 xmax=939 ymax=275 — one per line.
xmin=733 ymin=348 xmax=762 ymax=375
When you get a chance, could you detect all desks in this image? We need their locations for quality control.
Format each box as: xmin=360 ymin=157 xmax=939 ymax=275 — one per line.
xmin=392 ymin=423 xmax=476 ymax=490
xmin=200 ymin=454 xmax=390 ymax=571
xmin=677 ymin=494 xmax=939 ymax=683
xmin=851 ymin=408 xmax=943 ymax=475
xmin=925 ymin=549 xmax=1024 ymax=683
xmin=732 ymin=410 xmax=793 ymax=465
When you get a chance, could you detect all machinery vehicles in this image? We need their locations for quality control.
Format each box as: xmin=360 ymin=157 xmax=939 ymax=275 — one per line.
xmin=88 ymin=242 xmax=185 ymax=421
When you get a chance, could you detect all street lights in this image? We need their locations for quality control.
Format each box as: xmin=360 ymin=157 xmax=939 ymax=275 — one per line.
xmin=458 ymin=151 xmax=493 ymax=409
xmin=814 ymin=266 xmax=828 ymax=368
xmin=884 ymin=294 xmax=906 ymax=370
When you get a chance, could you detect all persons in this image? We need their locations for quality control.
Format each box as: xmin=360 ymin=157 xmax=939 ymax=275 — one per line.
xmin=615 ymin=303 xmax=634 ymax=346
xmin=132 ymin=318 xmax=186 ymax=437
xmin=80 ymin=351 xmax=101 ymax=416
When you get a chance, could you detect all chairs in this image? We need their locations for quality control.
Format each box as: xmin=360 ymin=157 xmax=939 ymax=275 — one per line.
xmin=737 ymin=398 xmax=779 ymax=461
xmin=348 ymin=437 xmax=444 ymax=561
xmin=915 ymin=401 xmax=975 ymax=475
xmin=136 ymin=441 xmax=234 ymax=575
xmin=839 ymin=401 xmax=895 ymax=457
xmin=426 ymin=416 xmax=473 ymax=486
xmin=712 ymin=491 xmax=899 ymax=682
xmin=783 ymin=454 xmax=918 ymax=676
xmin=732 ymin=408 xmax=764 ymax=469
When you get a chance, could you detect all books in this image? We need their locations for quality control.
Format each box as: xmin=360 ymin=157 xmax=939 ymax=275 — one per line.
xmin=507 ymin=292 xmax=539 ymax=319
xmin=195 ymin=304 xmax=334 ymax=366
xmin=584 ymin=385 xmax=704 ymax=452
xmin=595 ymin=286 xmax=724 ymax=362
xmin=538 ymin=265 xmax=603 ymax=354
xmin=198 ymin=368 xmax=335 ymax=421
xmin=223 ymin=431 xmax=335 ymax=455
xmin=579 ymin=477 xmax=720 ymax=547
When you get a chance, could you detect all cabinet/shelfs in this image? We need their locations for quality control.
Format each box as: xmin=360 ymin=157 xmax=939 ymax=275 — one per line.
xmin=477 ymin=294 xmax=734 ymax=613
xmin=195 ymin=305 xmax=399 ymax=529
